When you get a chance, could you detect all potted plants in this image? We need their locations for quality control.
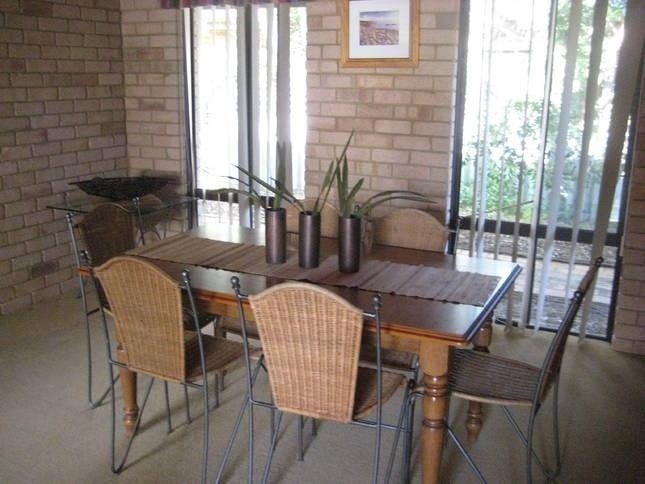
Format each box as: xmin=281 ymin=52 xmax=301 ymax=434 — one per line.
xmin=206 ymin=125 xmax=440 ymax=276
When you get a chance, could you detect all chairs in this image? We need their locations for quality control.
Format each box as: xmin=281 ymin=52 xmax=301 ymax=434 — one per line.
xmin=79 ymin=244 xmax=262 ymax=484
xmin=66 ymin=199 xmax=219 ymax=410
xmin=383 ymin=256 xmax=605 ymax=483
xmin=354 ymin=196 xmax=452 ymax=254
xmin=279 ymin=196 xmax=344 ymax=240
xmin=231 ymin=273 xmax=417 ymax=483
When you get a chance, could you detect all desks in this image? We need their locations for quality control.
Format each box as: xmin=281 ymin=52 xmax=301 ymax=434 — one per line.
xmin=42 ymin=196 xmax=199 ymax=297
xmin=77 ymin=229 xmax=526 ymax=484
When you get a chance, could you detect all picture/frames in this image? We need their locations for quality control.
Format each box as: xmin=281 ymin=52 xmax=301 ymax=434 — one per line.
xmin=338 ymin=0 xmax=421 ymax=69
